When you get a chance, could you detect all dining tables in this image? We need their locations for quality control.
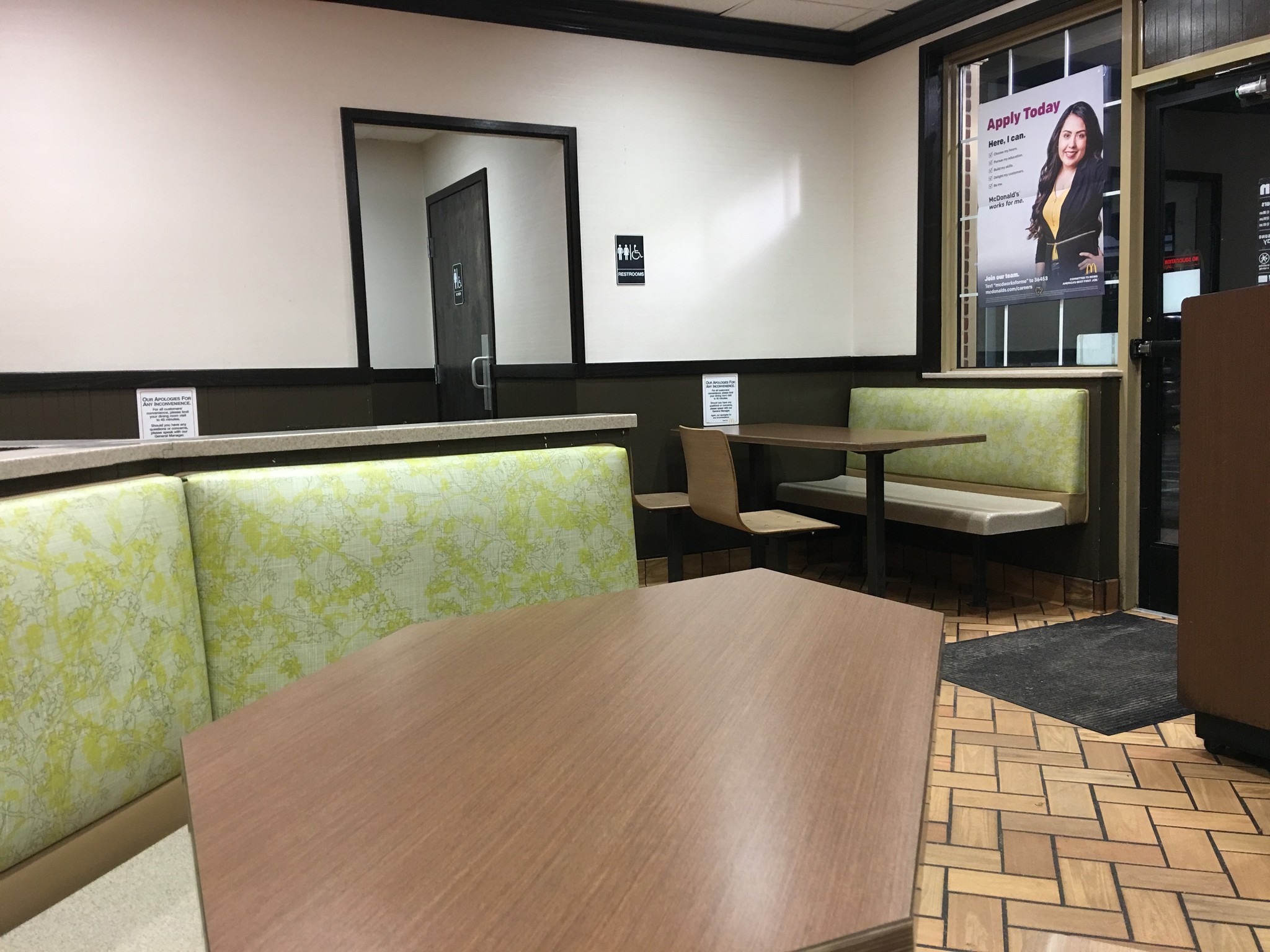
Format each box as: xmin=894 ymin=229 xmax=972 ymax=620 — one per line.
xmin=669 ymin=424 xmax=986 ymax=599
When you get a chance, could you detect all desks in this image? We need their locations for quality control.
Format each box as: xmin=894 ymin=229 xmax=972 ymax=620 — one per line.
xmin=1175 ymin=280 xmax=1270 ymax=777
xmin=1 ymin=417 xmax=633 ymax=952
xmin=171 ymin=558 xmax=945 ymax=950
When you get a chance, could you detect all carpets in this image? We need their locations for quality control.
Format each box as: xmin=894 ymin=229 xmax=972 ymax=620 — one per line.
xmin=932 ymin=609 xmax=1196 ymax=736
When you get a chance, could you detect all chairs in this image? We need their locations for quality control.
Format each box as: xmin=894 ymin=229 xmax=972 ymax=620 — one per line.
xmin=627 ymin=442 xmax=700 ymax=589
xmin=676 ymin=424 xmax=841 ymax=587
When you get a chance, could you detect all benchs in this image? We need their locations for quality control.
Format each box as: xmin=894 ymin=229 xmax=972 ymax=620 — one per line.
xmin=775 ymin=384 xmax=1089 ymax=605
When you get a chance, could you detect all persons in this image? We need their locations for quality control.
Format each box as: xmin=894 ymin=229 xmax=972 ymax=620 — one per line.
xmin=1026 ymin=101 xmax=1104 ymax=302
